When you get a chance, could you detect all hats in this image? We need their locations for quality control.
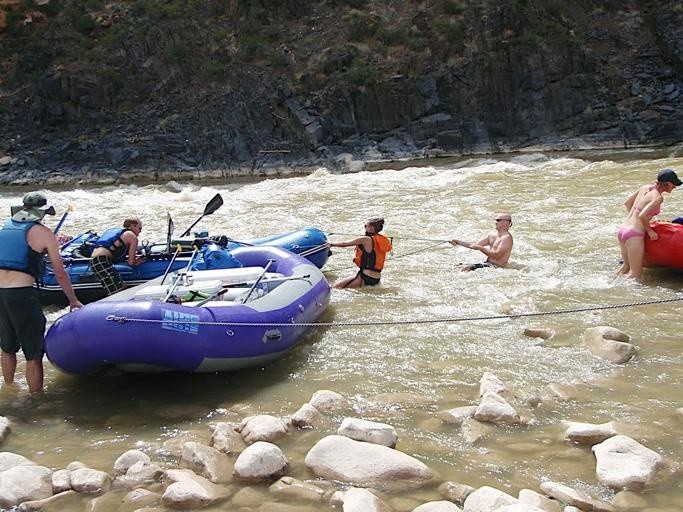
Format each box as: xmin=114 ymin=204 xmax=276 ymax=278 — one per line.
xmin=11 ymin=192 xmax=56 ymax=222
xmin=657 ymin=170 xmax=683 ymax=186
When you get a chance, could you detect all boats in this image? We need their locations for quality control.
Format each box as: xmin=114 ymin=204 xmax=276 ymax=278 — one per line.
xmin=34 ymin=227 xmax=332 ymax=306
xmin=642 ymin=219 xmax=683 ymax=270
xmin=45 ymin=245 xmax=330 ymax=378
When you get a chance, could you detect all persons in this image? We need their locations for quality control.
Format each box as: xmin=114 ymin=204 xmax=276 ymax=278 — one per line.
xmin=449 ymin=213 xmax=514 ymax=275
xmin=0 ymin=192 xmax=84 ymax=392
xmin=330 ymin=218 xmax=393 ymax=292
xmin=613 ymin=169 xmax=683 ymax=282
xmin=90 ymin=217 xmax=146 ymax=297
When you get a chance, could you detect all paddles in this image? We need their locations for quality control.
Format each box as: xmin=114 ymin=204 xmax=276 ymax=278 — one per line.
xmin=179 ymin=193 xmax=224 ymax=238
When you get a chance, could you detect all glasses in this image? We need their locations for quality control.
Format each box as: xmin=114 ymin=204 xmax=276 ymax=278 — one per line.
xmin=365 ymin=224 xmax=370 ymax=228
xmin=496 ymin=218 xmax=507 ymax=222
